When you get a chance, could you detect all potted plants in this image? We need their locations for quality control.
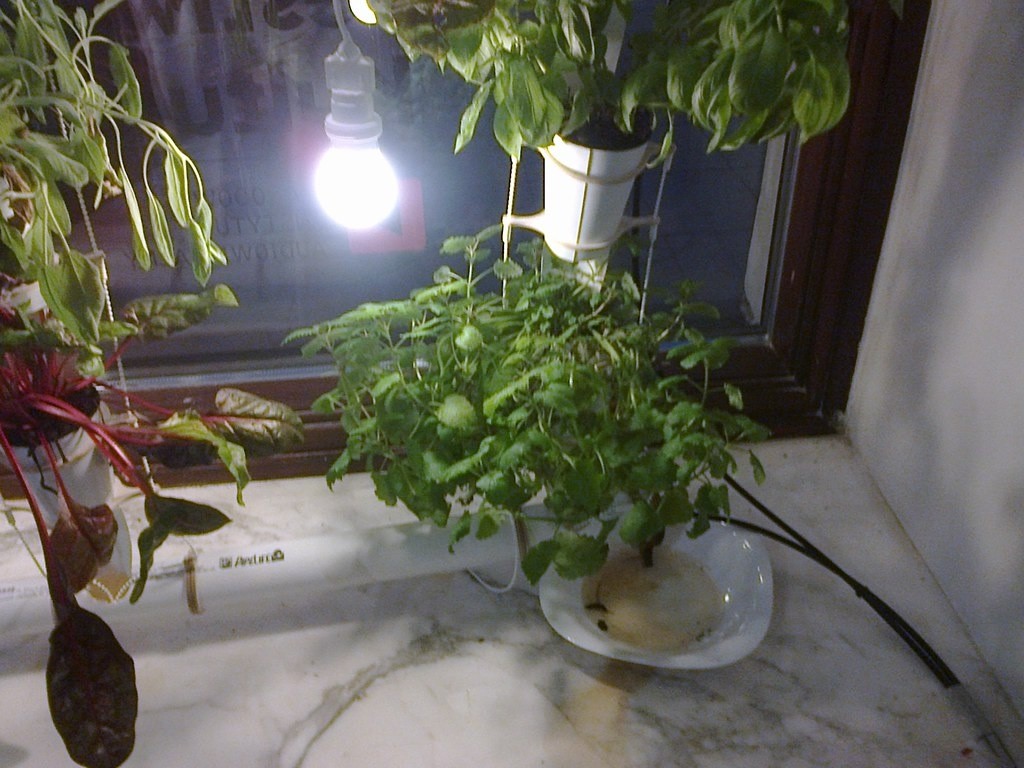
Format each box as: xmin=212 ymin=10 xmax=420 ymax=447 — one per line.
xmin=0 ymin=0 xmax=305 ymax=768
xmin=278 ymin=0 xmax=854 ymax=582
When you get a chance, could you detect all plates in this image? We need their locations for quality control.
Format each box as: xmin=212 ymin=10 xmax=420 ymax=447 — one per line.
xmin=541 ymin=511 xmax=775 ymax=669
